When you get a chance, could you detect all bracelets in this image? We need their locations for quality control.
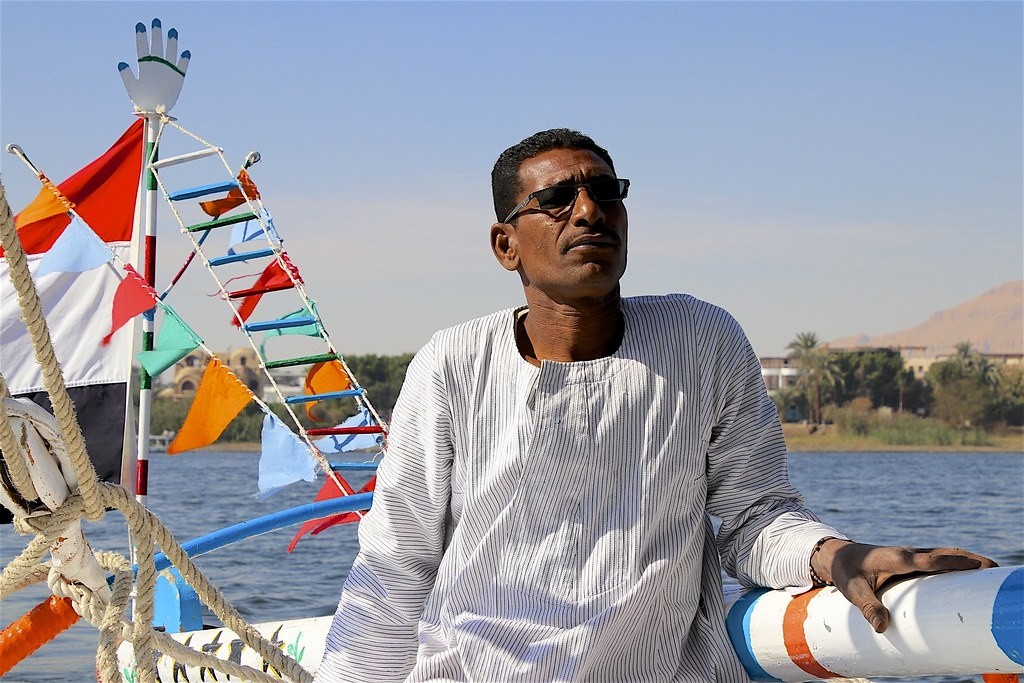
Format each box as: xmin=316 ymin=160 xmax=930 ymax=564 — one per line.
xmin=810 ymin=538 xmax=854 ymax=587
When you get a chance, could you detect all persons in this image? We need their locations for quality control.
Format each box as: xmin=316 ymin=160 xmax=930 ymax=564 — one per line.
xmin=315 ymin=127 xmax=999 ymax=683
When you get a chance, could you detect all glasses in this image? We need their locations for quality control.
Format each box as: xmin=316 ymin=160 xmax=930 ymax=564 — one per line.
xmin=504 ymin=178 xmax=630 ymax=224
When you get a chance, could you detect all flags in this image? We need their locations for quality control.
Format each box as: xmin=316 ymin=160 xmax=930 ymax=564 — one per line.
xmin=0 ymin=117 xmax=145 ymax=523
